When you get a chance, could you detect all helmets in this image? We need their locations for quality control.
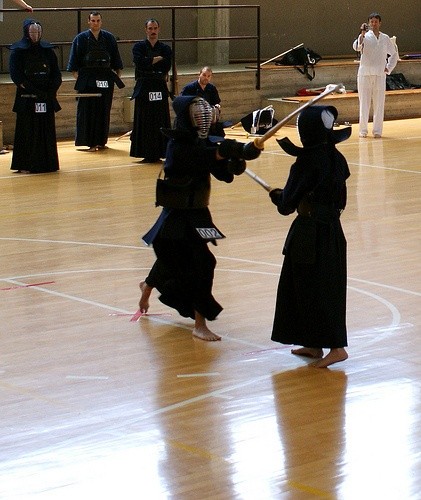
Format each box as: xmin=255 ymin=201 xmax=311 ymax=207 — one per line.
xmin=23 ymin=19 xmax=43 ymax=44
xmin=297 ymin=105 xmax=338 ymax=146
xmin=172 ymin=96 xmax=214 ymax=139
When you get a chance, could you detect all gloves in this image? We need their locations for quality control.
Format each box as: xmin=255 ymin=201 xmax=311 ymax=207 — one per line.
xmin=269 ymin=188 xmax=285 ymax=205
xmin=242 ymin=141 xmax=261 ymax=160
xmin=224 ymin=155 xmax=246 ymax=176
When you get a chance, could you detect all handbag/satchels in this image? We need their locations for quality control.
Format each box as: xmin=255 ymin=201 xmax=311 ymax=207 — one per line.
xmin=280 ymin=46 xmax=322 ymax=80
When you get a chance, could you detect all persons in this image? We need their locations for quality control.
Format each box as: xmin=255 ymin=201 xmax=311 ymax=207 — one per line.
xmin=66 ymin=11 xmax=123 ymax=152
xmin=137 ymin=94 xmax=265 ymax=341
xmin=131 ymin=17 xmax=174 ymax=162
xmin=263 ymin=102 xmax=350 ymax=370
xmin=8 ymin=17 xmax=63 ymax=174
xmin=350 ymin=12 xmax=399 ymax=138
xmin=179 ymin=65 xmax=225 ymax=138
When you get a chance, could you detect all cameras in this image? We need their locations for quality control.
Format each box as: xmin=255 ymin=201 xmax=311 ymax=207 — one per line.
xmin=364 ymin=25 xmax=374 ymax=33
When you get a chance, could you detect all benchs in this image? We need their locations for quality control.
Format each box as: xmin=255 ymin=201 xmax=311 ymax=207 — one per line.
xmin=264 ymin=89 xmax=421 ymax=125
